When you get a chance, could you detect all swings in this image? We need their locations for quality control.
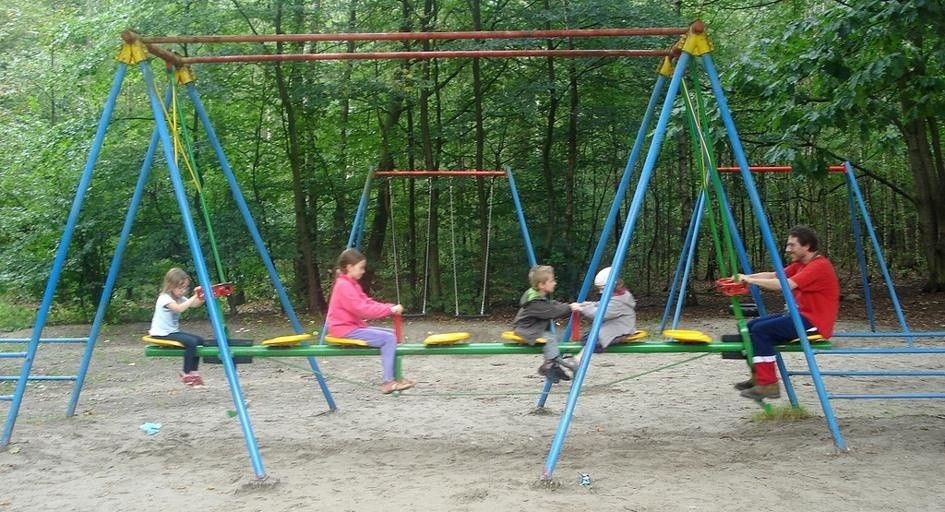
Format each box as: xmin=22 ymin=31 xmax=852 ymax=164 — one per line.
xmin=387 ymin=175 xmax=432 ymax=317
xmin=448 ymin=175 xmax=494 ymax=318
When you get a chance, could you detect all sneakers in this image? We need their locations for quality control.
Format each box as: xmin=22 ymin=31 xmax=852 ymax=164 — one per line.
xmin=181 ymin=374 xmax=205 ymax=388
xmin=735 ymin=378 xmax=781 ymax=400
xmin=538 ymin=360 xmax=571 ymax=383
xmin=381 ymin=377 xmax=418 ymax=395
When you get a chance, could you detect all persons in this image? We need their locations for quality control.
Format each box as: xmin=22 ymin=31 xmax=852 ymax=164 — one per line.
xmin=728 ymin=226 xmax=842 ymax=401
xmin=323 ymin=247 xmax=417 ymax=395
xmin=148 ymin=267 xmax=208 ymax=390
xmin=511 ymin=264 xmax=583 ymax=386
xmin=556 ymin=266 xmax=637 ymax=373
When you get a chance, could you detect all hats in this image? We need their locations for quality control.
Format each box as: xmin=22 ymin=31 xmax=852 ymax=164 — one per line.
xmin=593 ymin=266 xmax=613 ymax=288
